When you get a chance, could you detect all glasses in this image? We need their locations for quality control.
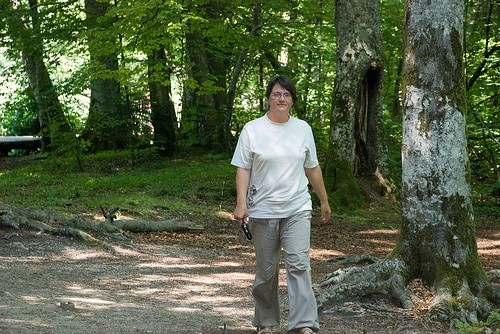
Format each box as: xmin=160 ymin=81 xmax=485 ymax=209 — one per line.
xmin=270 ymin=93 xmax=293 ymax=98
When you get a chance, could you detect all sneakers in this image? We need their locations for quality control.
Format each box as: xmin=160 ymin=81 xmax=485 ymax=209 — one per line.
xmin=256 ymin=326 xmax=272 ymax=334
xmin=298 ymin=328 xmax=315 ymax=334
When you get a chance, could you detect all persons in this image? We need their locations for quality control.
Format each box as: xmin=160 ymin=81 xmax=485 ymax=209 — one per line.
xmin=230 ymin=76 xmax=331 ymax=334
